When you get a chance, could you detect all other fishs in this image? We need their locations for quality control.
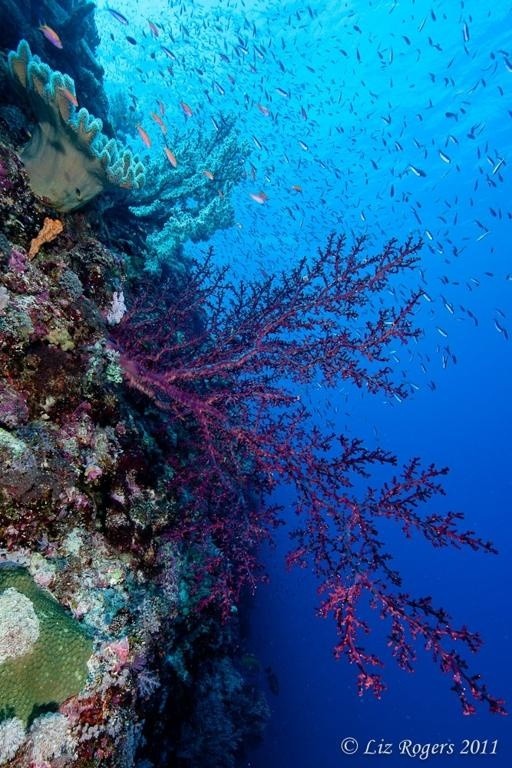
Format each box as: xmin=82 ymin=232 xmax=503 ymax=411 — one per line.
xmin=36 ymin=0 xmax=511 ymax=441
